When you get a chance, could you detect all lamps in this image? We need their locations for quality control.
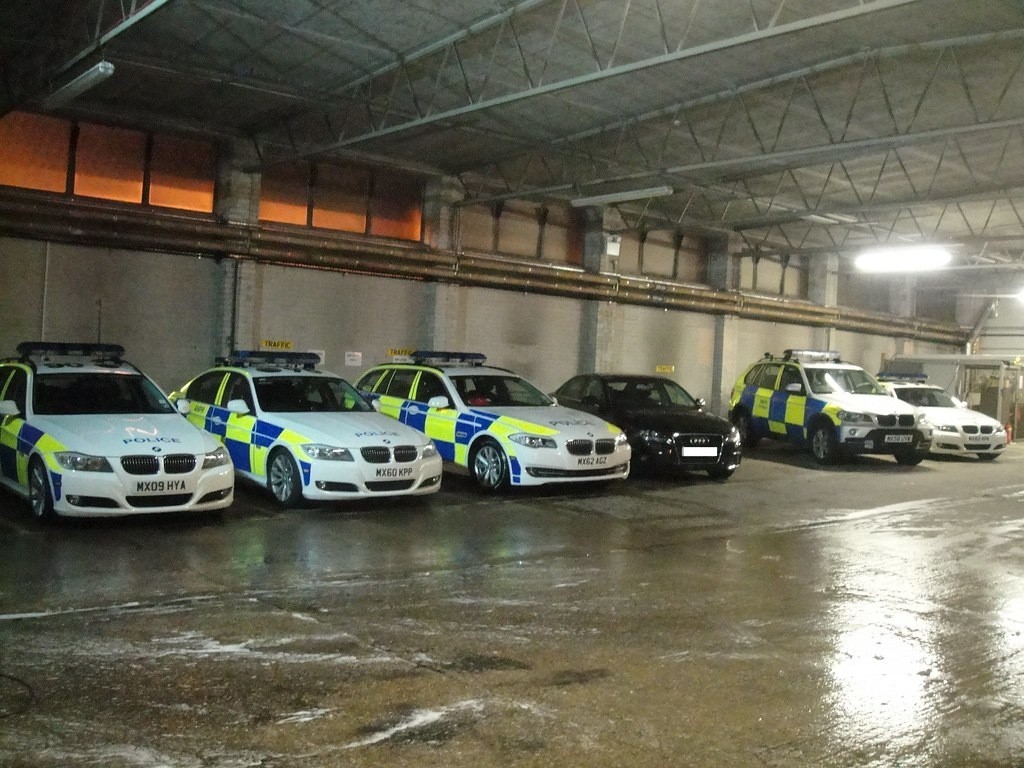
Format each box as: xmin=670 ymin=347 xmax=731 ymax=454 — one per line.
xmin=571 ymin=186 xmax=673 ymax=208
xmin=45 ymin=59 xmax=115 ymax=108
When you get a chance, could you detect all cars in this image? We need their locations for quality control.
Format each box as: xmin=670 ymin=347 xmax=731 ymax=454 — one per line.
xmin=548 ymin=372 xmax=743 ymax=483
xmin=352 ymin=349 xmax=631 ymax=494
xmin=1 ymin=340 xmax=236 ymax=528
xmin=165 ymin=350 xmax=444 ymax=509
xmin=852 ymin=371 xmax=1008 ymax=459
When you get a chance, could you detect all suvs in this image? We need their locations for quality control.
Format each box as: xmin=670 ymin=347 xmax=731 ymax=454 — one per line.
xmin=728 ymin=350 xmax=933 ymax=467
